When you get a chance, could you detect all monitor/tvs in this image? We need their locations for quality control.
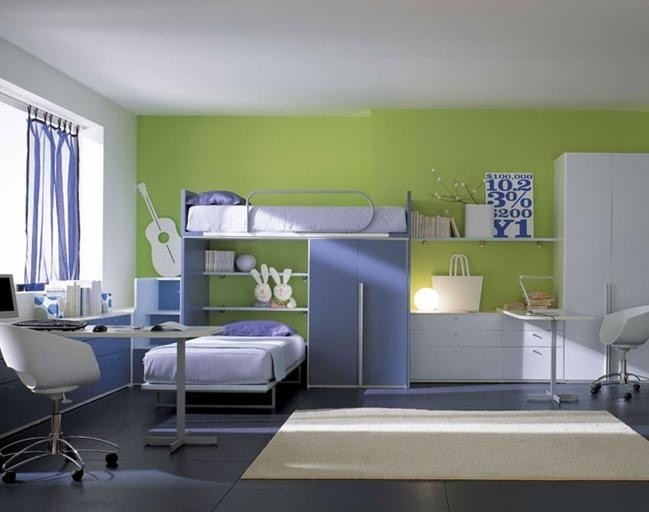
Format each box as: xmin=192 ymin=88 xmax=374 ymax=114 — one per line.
xmin=0 ymin=274 xmax=19 ymax=325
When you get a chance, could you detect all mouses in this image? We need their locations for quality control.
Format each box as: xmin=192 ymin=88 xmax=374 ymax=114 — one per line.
xmin=93 ymin=324 xmax=107 ymax=332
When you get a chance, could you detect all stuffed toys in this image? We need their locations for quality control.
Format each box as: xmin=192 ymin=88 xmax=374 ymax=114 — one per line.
xmin=250 ymin=264 xmax=272 ymax=307
xmin=270 ymin=267 xmax=297 ymax=308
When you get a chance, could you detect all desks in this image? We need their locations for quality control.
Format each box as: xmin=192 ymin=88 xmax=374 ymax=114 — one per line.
xmin=502 ymin=309 xmax=597 ymax=407
xmin=9 ymin=321 xmax=227 ymax=452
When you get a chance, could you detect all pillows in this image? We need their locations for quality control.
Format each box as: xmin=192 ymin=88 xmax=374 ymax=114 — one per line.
xmin=213 ymin=320 xmax=297 ymax=336
xmin=186 ymin=190 xmax=251 ymax=205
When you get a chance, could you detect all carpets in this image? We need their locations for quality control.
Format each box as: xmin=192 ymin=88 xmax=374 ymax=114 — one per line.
xmin=240 ymin=407 xmax=649 ymax=481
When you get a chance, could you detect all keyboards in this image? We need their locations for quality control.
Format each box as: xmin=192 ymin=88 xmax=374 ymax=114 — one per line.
xmin=12 ymin=319 xmax=89 ymax=331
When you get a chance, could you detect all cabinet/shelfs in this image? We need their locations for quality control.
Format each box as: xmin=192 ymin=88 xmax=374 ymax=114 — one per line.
xmin=551 ymin=151 xmax=649 ymax=384
xmin=202 ymin=271 xmax=308 ymax=312
xmin=410 ymin=312 xmax=564 ymax=383
xmin=131 ymin=277 xmax=180 ymax=386
xmin=308 ymin=237 xmax=409 ymax=389
xmin=0 ymin=307 xmax=134 ymax=438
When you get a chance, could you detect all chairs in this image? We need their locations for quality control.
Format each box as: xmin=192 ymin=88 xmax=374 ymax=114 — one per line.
xmin=589 ymin=305 xmax=649 ymax=399
xmin=0 ymin=323 xmax=120 ymax=481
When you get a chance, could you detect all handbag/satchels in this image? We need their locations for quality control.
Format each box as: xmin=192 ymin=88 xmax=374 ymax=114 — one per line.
xmin=434 ymin=275 xmax=484 ymax=312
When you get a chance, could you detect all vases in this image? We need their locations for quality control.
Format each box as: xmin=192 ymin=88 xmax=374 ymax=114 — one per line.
xmin=464 ymin=204 xmax=494 ymax=237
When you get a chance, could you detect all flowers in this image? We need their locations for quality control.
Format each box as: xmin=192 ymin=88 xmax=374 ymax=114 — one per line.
xmin=429 ymin=168 xmax=485 ymax=214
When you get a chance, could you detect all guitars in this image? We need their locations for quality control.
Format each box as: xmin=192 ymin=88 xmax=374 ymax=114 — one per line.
xmin=139 ymin=184 xmax=181 ymax=278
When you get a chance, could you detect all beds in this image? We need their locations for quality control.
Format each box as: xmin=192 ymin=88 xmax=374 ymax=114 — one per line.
xmin=186 ymin=205 xmax=406 ymax=233
xmin=142 ymin=334 xmax=305 ymax=409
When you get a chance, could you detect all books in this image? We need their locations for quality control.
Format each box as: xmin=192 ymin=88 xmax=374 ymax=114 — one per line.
xmin=504 ymin=291 xmax=555 ymax=309
xmin=411 ymin=210 xmax=459 ymax=237
xmin=47 ymin=276 xmax=102 ymax=317
xmin=203 ymin=250 xmax=236 ymax=272
